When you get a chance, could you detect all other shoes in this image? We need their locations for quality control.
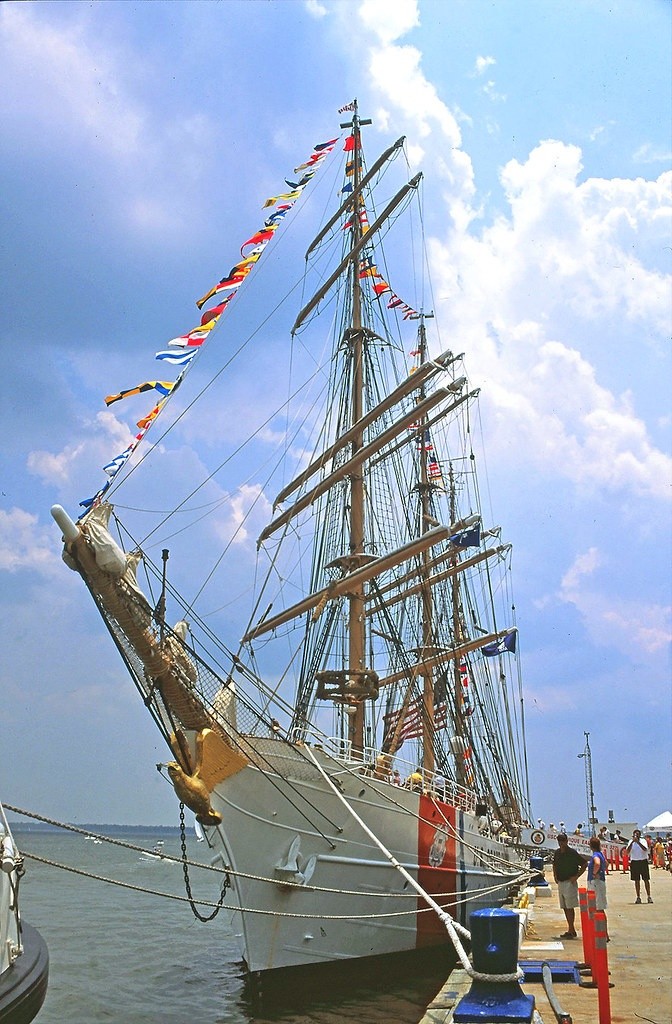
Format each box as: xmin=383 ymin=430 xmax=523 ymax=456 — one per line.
xmin=647 ymin=897 xmax=653 ymax=903
xmin=635 ymin=897 xmax=642 ymax=904
xmin=560 ymin=932 xmax=578 ymax=938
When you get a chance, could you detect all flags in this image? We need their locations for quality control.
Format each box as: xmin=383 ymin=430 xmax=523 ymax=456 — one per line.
xmin=386 ymin=293 xmax=417 ymax=320
xmin=340 ymin=190 xmax=388 ymax=294
xmin=407 ymin=348 xmax=442 ymax=481
xmin=381 ymin=668 xmax=449 ymax=753
xmin=463 ymin=692 xmax=476 ymax=785
xmin=449 ymin=522 xmax=481 ymax=547
xmin=338 ymin=101 xmax=354 ymax=114
xmin=481 ymin=630 xmax=517 ymax=657
xmin=460 ymin=663 xmax=467 ymax=687
xmin=345 ymin=157 xmax=363 ymax=177
xmin=343 ymin=131 xmax=361 ymax=152
xmin=240 ymin=170 xmax=316 ymax=258
xmin=78 ymin=380 xmax=180 ymax=506
xmin=294 ymin=137 xmax=339 ymax=174
xmin=156 ymin=254 xmax=262 ymax=364
xmin=337 ymin=181 xmax=353 ymax=198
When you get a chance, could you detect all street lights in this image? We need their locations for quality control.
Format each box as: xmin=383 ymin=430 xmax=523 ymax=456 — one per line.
xmin=577 ymin=731 xmax=596 ymax=838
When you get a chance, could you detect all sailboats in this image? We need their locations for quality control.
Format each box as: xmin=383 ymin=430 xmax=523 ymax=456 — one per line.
xmin=48 ymin=94 xmax=535 ymax=980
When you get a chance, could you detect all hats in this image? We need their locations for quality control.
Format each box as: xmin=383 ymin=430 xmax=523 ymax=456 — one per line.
xmin=557 ymin=832 xmax=567 ymax=840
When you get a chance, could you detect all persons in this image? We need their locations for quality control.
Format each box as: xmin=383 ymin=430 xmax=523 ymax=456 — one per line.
xmin=550 ymin=821 xmax=621 ymax=843
xmin=587 ymin=838 xmax=610 ymax=942
xmin=626 ymin=830 xmax=653 ymax=903
xmin=392 ymin=768 xmax=445 ymax=801
xmin=552 ymin=833 xmax=588 ymax=938
xmin=453 ymin=789 xmax=546 ymax=837
xmin=645 ymin=833 xmax=672 ymax=873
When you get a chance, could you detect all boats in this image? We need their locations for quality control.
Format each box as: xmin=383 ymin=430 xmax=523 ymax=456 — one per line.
xmin=157 ymin=839 xmax=164 ymax=846
xmin=93 ymin=838 xmax=102 ymax=844
xmin=0 ymin=801 xmax=51 ymax=1024
xmin=84 ymin=834 xmax=96 ymax=840
